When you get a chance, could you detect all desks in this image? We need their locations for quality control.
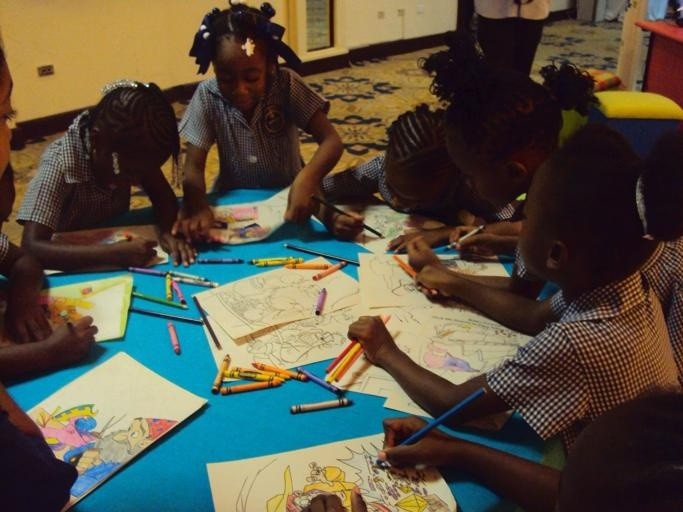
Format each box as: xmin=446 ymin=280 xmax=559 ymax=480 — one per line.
xmin=635 ymin=20 xmax=683 ymax=106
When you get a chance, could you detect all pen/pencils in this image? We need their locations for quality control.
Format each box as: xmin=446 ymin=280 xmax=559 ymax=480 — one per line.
xmin=310 ymin=194 xmax=386 ymax=239
xmin=122 ymin=232 xmax=136 ymax=243
xmin=211 ymin=316 xmax=398 ymax=413
xmin=129 ymin=258 xmax=244 ymax=354
xmin=444 ymin=225 xmax=484 ymax=252
xmin=373 ymin=387 xmax=487 ymax=469
xmin=392 ymin=255 xmax=438 ymax=295
xmin=60 ymin=310 xmax=73 ymax=325
xmin=248 ymin=243 xmax=360 ymax=315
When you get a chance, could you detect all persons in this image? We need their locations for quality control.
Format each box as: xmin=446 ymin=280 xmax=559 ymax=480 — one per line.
xmin=301 ymin=387 xmax=682 ymax=512
xmin=351 ymin=126 xmax=682 ymax=459
xmin=167 ymin=1 xmax=345 ymax=244
xmin=0 ymin=42 xmax=77 ymax=512
xmin=317 ymin=94 xmax=526 ymax=256
xmin=473 ymin=0 xmax=550 ymax=77
xmin=395 ymin=26 xmax=682 ymax=383
xmin=13 ymin=80 xmax=197 ymax=270
xmin=0 ymin=161 xmax=104 ymax=379
xmin=61 ymin=413 xmax=177 ymax=499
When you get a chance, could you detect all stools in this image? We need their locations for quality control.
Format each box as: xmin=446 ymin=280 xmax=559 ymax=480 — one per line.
xmin=592 ymin=91 xmax=682 ymax=160
xmin=582 ymin=67 xmax=625 ymax=91
